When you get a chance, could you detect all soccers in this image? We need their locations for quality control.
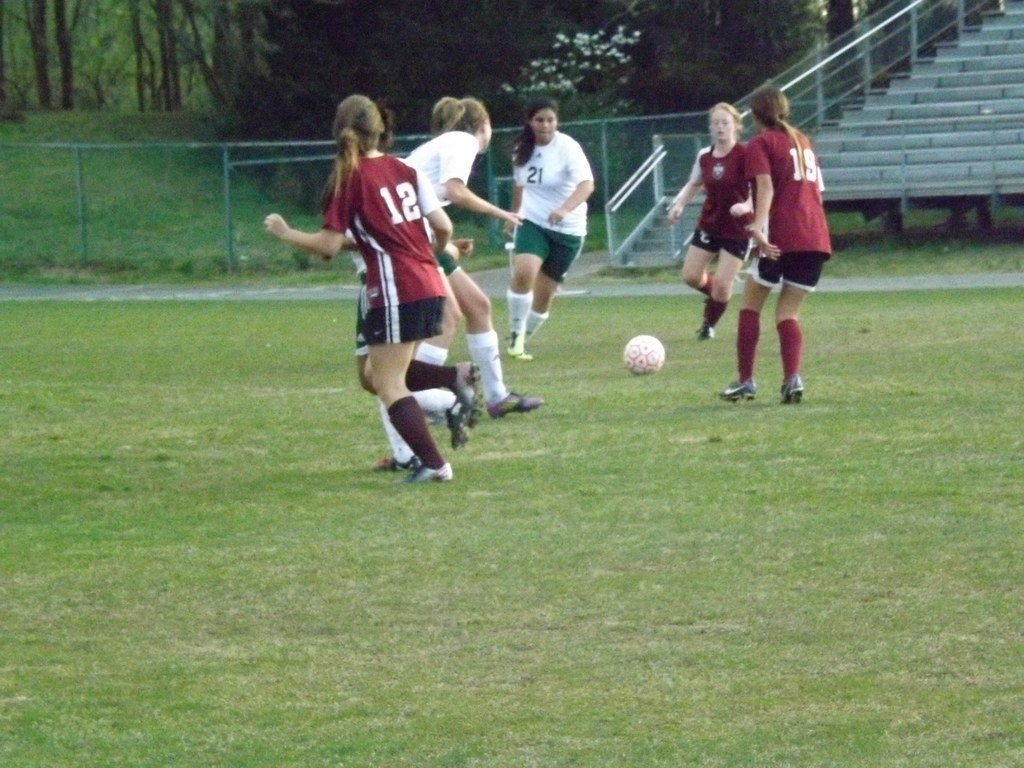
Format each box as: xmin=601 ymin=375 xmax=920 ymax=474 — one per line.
xmin=622 ymin=333 xmax=668 ymax=377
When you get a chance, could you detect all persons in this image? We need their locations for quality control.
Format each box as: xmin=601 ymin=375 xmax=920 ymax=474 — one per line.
xmin=504 ymin=98 xmax=595 ymax=361
xmin=402 ymin=96 xmax=543 ymax=417
xmin=669 ymin=102 xmax=757 ymax=340
xmin=720 ymin=85 xmax=831 ymax=404
xmin=264 ymin=95 xmax=484 ymax=484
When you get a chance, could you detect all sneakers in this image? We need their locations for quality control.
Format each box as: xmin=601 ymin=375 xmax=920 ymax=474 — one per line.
xmin=697 ymin=322 xmax=714 ymax=340
xmin=712 ymin=379 xmax=758 ymax=402
xmin=486 ymin=391 xmax=546 ymax=419
xmin=780 ymin=373 xmax=803 ymax=404
xmin=452 ymin=361 xmax=484 ymax=430
xmin=507 ymin=321 xmax=535 ymax=363
xmin=405 ymin=462 xmax=453 ymax=482
xmin=373 ymin=454 xmax=423 ymax=471
xmin=445 ymin=396 xmax=475 ymax=452
